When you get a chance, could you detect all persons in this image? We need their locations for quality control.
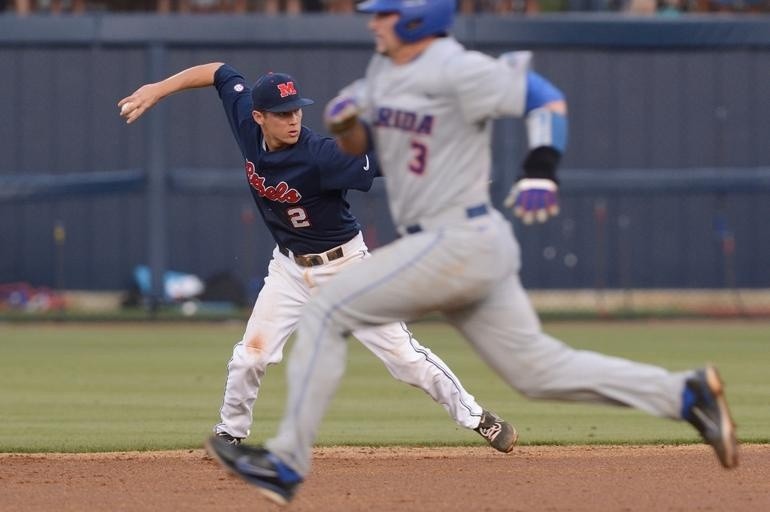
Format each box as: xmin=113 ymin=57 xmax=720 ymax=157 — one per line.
xmin=206 ymin=0 xmax=739 ymax=507
xmin=116 ymin=62 xmax=519 ymax=453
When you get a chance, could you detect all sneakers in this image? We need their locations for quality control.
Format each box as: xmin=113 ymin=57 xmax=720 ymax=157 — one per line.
xmin=207 ymin=432 xmax=303 ymax=508
xmin=473 ymin=408 xmax=518 ymax=452
xmin=683 ymin=364 xmax=736 ymax=469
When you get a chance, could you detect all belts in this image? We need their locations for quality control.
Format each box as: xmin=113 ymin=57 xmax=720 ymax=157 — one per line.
xmin=279 ymin=245 xmax=343 ymax=268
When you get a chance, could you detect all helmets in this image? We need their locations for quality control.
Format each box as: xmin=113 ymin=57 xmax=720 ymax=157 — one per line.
xmin=355 ymin=0 xmax=458 ymax=42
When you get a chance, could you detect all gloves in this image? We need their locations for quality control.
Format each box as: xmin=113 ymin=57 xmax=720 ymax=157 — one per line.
xmin=324 ymin=95 xmax=361 ymax=134
xmin=502 ymin=167 xmax=560 ymax=225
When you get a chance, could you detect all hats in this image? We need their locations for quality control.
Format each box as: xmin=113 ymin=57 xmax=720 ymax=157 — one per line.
xmin=252 ymin=72 xmax=314 ymax=112
